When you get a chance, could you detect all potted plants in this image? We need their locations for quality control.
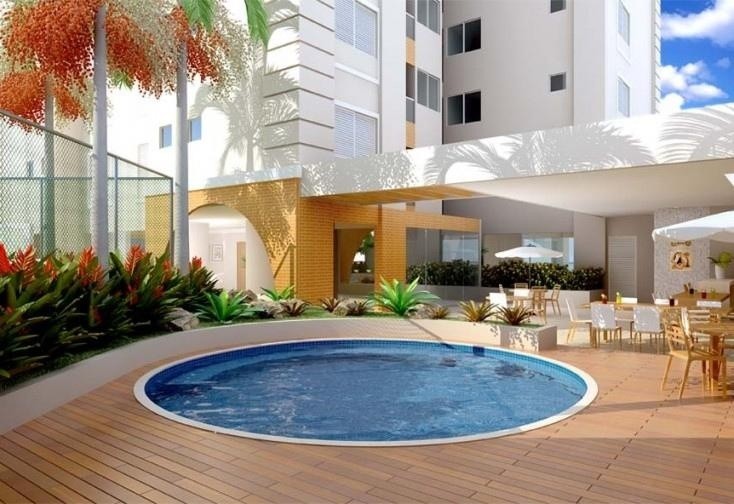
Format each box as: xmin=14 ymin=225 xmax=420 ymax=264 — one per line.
xmin=707 ymin=250 xmax=733 ymax=279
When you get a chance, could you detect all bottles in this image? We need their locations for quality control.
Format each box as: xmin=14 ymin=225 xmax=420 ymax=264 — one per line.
xmin=614 ymin=291 xmax=621 ymax=305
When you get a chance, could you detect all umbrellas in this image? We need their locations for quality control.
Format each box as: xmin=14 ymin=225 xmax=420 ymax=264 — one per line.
xmin=494 ymin=243 xmax=563 ymax=289
xmin=652 ymin=210 xmax=734 ymax=243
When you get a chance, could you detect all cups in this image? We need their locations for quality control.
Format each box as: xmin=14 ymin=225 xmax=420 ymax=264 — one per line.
xmin=669 ymin=286 xmax=715 ymax=307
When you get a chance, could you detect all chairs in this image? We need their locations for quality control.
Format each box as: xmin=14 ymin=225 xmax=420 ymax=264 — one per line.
xmin=497 ymin=281 xmax=734 ymax=402
